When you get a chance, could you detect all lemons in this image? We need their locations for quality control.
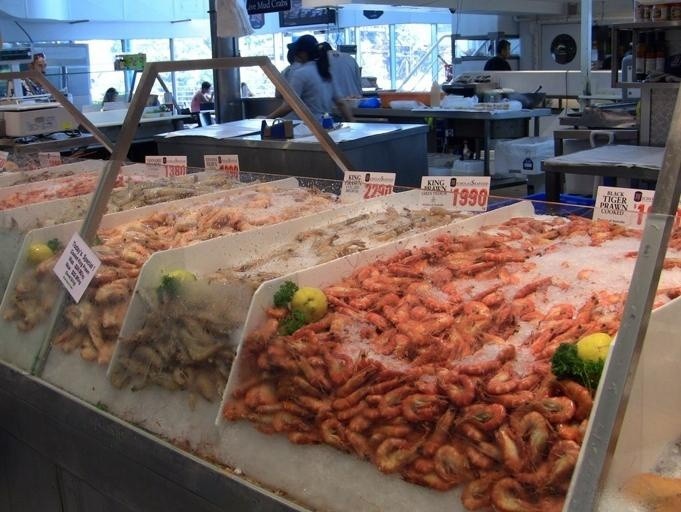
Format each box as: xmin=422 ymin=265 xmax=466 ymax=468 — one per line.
xmin=169 ymin=269 xmax=197 ymax=283
xmin=26 ymin=243 xmax=53 ymax=264
xmin=291 ymin=286 xmax=328 ymax=322
xmin=576 ymin=332 xmax=613 ymax=363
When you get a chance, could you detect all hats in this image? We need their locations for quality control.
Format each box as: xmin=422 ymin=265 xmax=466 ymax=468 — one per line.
xmin=288 ymin=35 xmax=317 ymax=49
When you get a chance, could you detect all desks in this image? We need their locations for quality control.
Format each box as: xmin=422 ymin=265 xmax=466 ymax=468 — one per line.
xmin=542 ymin=144 xmax=663 ymax=202
xmin=154 ymin=118 xmax=428 ymax=187
xmin=332 ymin=109 xmax=552 ymax=195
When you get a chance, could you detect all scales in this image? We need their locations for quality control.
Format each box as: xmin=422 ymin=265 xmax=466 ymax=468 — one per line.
xmin=0 ymin=48 xmax=50 ymax=104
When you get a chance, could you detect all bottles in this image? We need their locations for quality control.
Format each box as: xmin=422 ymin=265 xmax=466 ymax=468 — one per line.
xmin=636 ymin=41 xmax=666 ymax=81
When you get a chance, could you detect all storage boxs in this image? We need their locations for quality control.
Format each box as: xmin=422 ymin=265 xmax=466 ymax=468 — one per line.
xmin=492 ymin=137 xmax=554 ymax=175
xmin=0 ymin=104 xmax=79 ymax=138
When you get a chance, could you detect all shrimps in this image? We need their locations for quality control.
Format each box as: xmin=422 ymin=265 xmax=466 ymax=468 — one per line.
xmin=0 ymin=162 xmax=681 ymax=512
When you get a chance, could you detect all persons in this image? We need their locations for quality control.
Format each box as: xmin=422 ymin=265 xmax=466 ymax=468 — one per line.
xmin=484 ymin=40 xmax=512 ymax=71
xmin=21 ymin=53 xmax=46 ymax=95
xmin=102 ymin=88 xmax=119 ymax=106
xmin=191 ymin=82 xmax=215 ymax=127
xmin=266 ymin=35 xmax=362 ymax=121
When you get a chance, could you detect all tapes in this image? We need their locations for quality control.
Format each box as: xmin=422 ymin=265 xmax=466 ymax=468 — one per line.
xmin=272 ymin=118 xmax=285 ymax=125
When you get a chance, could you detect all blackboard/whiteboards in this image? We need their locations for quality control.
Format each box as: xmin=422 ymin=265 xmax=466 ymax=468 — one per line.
xmin=248 ymin=0 xmax=336 ymax=27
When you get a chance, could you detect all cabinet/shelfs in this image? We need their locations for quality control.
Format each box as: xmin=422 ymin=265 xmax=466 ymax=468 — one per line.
xmin=612 ymin=0 xmax=680 ymax=89
